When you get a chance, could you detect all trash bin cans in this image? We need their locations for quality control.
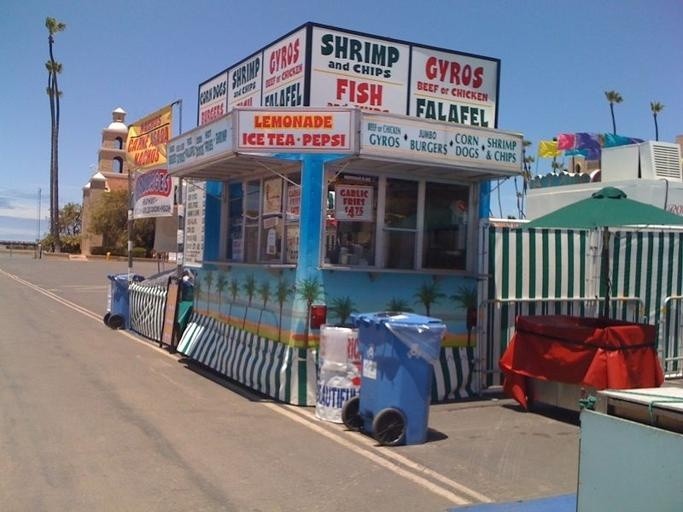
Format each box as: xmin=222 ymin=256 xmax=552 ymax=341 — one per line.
xmin=341 ymin=310 xmax=446 ymax=445
xmin=103 ymin=274 xmax=144 ymax=330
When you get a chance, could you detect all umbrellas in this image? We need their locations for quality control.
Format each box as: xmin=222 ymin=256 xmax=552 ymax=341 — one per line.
xmin=511 ymin=183 xmax=683 ymax=316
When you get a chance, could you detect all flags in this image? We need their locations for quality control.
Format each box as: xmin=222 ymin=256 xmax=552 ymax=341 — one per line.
xmin=539 ymin=130 xmax=644 ymax=160
